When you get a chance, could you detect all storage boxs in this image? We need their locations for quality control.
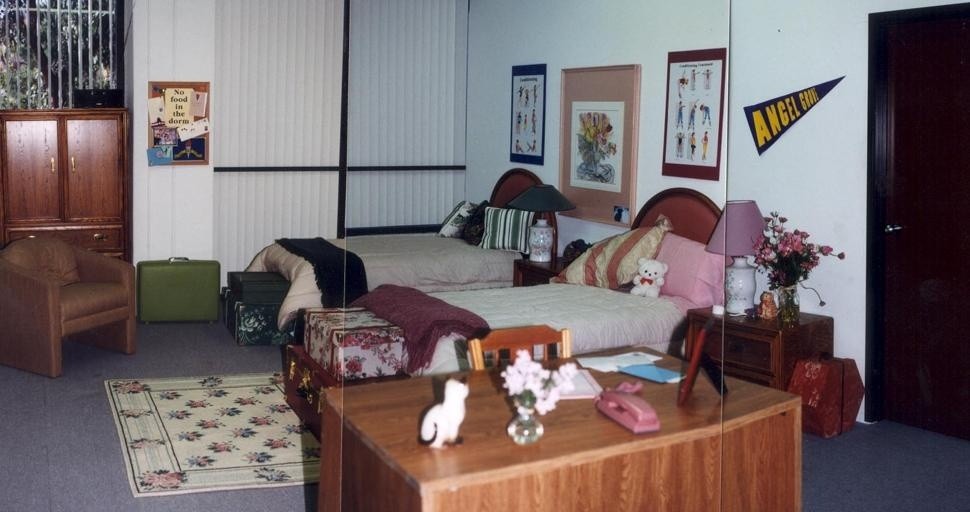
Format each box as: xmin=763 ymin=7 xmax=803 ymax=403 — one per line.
xmin=222 ymin=288 xmax=296 ymax=346
xmin=302 ymin=304 xmax=408 ymax=380
xmin=227 ymin=269 xmax=291 ymax=306
xmin=285 ymin=342 xmax=411 ymax=443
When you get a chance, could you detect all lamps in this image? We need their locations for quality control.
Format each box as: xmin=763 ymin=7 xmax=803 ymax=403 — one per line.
xmin=503 ymin=183 xmax=573 ymax=265
xmin=704 ymin=199 xmax=770 ymax=316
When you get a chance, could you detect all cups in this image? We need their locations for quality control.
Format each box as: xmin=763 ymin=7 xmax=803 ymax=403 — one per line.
xmin=712 ymin=304 xmax=725 ymax=317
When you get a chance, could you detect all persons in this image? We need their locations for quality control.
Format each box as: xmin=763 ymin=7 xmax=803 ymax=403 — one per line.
xmin=673 ymin=131 xmax=686 ymax=158
xmin=703 ymin=68 xmax=714 ymax=90
xmin=515 ymin=109 xmax=523 ymax=134
xmin=699 ymin=104 xmax=711 ymax=127
xmin=676 ymin=67 xmax=690 ymax=98
xmin=689 ymin=132 xmax=698 ymax=160
xmin=530 ymin=84 xmax=541 ymax=107
xmin=691 ymin=68 xmax=702 ymax=90
xmin=688 ymin=98 xmax=701 ymax=131
xmin=153 ymin=127 xmax=175 ymax=146
xmin=524 ymin=87 xmax=530 ymax=105
xmin=530 ymin=108 xmax=539 ymax=135
xmin=701 ymin=131 xmax=711 ymax=160
xmin=514 ymin=84 xmax=525 ymax=106
xmin=515 ymin=138 xmax=524 ymax=153
xmin=526 ymin=139 xmax=537 ymax=152
xmin=674 ymin=100 xmax=687 ymax=128
xmin=522 ymin=113 xmax=528 ymax=132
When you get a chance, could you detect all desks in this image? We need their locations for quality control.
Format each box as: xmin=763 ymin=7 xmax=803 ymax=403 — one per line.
xmin=317 ymin=346 xmax=804 ymax=511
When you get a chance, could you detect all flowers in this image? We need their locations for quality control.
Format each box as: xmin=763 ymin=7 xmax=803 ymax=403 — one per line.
xmin=749 ymin=206 xmax=845 ymax=315
xmin=501 ymin=346 xmax=568 ymax=418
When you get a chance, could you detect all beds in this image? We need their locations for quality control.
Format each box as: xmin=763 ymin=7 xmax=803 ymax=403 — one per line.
xmin=282 ymin=186 xmax=725 ymax=444
xmin=248 ymin=168 xmax=558 ymax=346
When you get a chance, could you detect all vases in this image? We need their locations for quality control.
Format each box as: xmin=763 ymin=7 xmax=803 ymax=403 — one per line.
xmin=781 ymin=279 xmax=801 ymax=323
xmin=504 ymin=398 xmax=547 ymax=445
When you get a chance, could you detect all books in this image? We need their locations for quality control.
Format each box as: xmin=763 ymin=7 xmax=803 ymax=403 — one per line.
xmin=616 ymin=364 xmax=688 ymax=384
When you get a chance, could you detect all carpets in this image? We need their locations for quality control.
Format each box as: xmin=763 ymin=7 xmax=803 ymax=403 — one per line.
xmin=102 ymin=368 xmax=327 ymax=498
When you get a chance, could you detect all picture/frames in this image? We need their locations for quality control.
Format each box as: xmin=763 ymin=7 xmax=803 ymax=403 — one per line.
xmin=675 ymin=326 xmax=730 ymax=404
xmin=558 ymin=64 xmax=637 ymax=230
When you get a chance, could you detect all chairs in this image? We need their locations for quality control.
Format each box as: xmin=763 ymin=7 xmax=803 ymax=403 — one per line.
xmin=1 ymin=234 xmax=140 ymax=377
xmin=466 ymin=319 xmax=572 ymax=371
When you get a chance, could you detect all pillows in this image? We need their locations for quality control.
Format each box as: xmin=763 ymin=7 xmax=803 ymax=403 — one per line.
xmin=464 ymin=198 xmax=494 ymax=246
xmin=549 ymin=214 xmax=670 ymax=292
xmin=652 ymin=227 xmax=728 ymax=310
xmin=439 ymin=198 xmax=476 ymax=239
xmin=480 ymin=202 xmax=535 ymax=254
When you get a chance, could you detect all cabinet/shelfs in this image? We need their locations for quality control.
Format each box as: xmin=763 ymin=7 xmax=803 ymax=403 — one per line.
xmin=5 ymin=107 xmax=124 ymax=258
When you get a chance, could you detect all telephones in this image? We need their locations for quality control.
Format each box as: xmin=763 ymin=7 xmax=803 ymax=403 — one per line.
xmin=596 ymin=391 xmax=661 ymax=433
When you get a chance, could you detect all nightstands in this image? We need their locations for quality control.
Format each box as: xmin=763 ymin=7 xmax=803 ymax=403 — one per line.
xmin=512 ymin=254 xmax=571 ymax=287
xmin=685 ymin=307 xmax=834 ymax=390
